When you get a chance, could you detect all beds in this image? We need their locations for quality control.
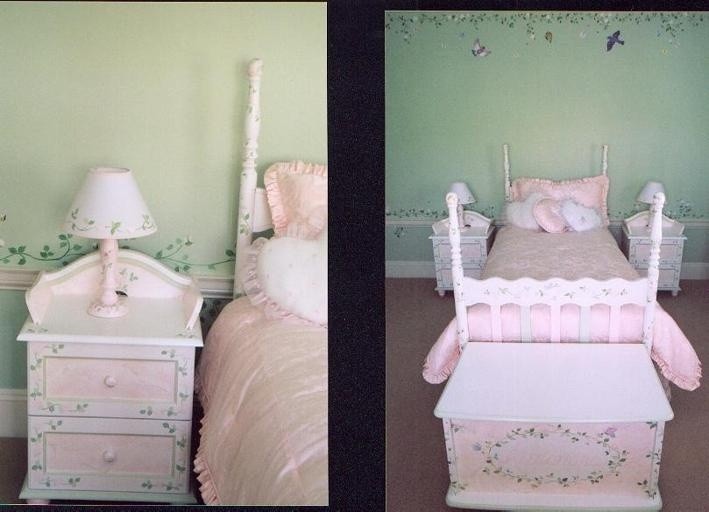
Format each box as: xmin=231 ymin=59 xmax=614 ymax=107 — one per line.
xmin=192 ymin=53 xmax=329 ymax=508
xmin=421 ymin=141 xmax=703 ymax=392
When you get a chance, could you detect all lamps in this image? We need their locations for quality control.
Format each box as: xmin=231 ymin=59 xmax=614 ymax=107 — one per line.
xmin=635 ymin=179 xmax=668 ymax=227
xmin=60 ymin=163 xmax=159 ymax=319
xmin=448 ymin=181 xmax=478 ymax=228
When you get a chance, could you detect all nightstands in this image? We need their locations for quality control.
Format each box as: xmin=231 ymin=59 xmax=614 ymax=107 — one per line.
xmin=621 ymin=209 xmax=687 ymax=298
xmin=427 ymin=209 xmax=497 ymax=296
xmin=15 ymin=245 xmax=210 ymax=507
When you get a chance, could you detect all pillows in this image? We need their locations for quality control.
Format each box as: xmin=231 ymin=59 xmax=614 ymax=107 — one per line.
xmin=503 ymin=174 xmax=614 ymax=233
xmin=240 ymin=233 xmax=329 ymax=329
xmin=261 ymin=157 xmax=327 ymax=239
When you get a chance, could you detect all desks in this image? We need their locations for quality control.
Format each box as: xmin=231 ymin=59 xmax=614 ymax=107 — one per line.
xmin=434 ymin=337 xmax=675 ymax=511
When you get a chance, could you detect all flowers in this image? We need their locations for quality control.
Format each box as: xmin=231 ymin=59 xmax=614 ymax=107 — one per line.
xmin=606 ymin=196 xmax=706 ymax=222
xmin=444 ymin=420 xmax=663 ymax=502
xmin=386 ymin=205 xmax=500 ymax=237
xmin=0 ymin=207 xmax=233 ymax=284
xmin=27 ymin=343 xmax=189 ymax=493
